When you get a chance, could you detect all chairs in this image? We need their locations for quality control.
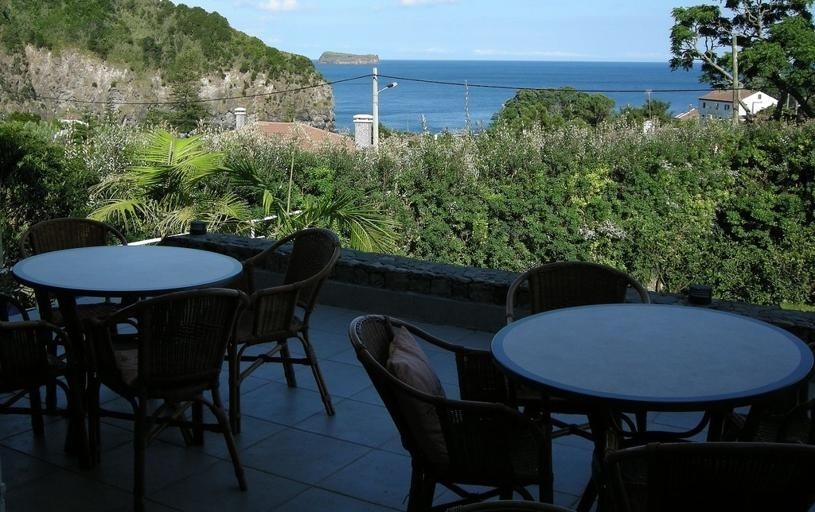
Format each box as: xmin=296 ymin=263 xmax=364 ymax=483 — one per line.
xmin=505 ymin=262 xmax=652 ymax=445
xmin=1 ymin=294 xmax=92 ymax=472
xmin=574 ymin=427 xmax=811 ymax=512
xmin=443 ymin=500 xmax=566 ymax=512
xmin=80 ymin=287 xmax=250 ymax=512
xmin=20 ymin=217 xmax=146 ymax=355
xmin=193 ymin=227 xmax=342 ymax=436
xmin=348 ymin=313 xmax=554 ymax=511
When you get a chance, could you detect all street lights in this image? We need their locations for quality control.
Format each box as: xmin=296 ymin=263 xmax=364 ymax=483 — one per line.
xmin=370 ymin=81 xmax=401 ymax=154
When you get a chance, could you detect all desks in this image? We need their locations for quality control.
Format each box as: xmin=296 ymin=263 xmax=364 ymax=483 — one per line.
xmin=490 ymin=303 xmax=810 ymax=511
xmin=13 ymin=247 xmax=243 ymax=445
